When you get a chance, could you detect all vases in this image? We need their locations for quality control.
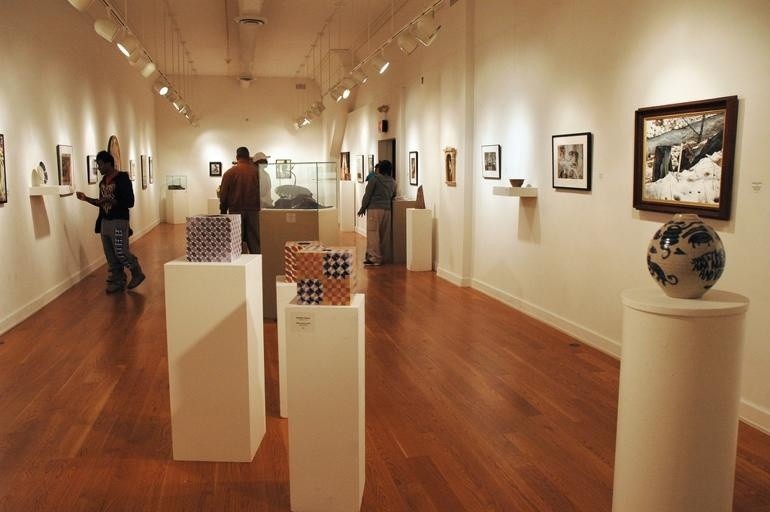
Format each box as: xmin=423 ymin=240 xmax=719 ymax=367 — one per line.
xmin=647 ymin=212 xmax=726 ymax=299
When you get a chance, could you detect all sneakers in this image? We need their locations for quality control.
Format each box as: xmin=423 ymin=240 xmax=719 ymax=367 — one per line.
xmin=105 ymin=280 xmax=126 ymax=294
xmin=363 ymin=259 xmax=381 ymax=266
xmin=127 ymin=273 xmax=145 ymax=289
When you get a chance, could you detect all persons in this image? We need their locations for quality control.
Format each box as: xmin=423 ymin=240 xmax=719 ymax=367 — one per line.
xmin=356 ymin=159 xmax=398 ymax=266
xmin=219 ymin=147 xmax=261 ymax=255
xmin=75 ymin=149 xmax=146 ymax=295
xmin=251 ymin=152 xmax=274 ymax=209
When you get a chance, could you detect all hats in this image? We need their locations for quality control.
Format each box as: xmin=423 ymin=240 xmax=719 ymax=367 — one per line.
xmin=253 ymin=152 xmax=267 ymax=163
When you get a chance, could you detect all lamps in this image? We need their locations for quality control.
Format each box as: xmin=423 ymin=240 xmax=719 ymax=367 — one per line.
xmin=289 ymin=1 xmax=459 ymax=131
xmin=68 ymin=0 xmax=200 ymax=129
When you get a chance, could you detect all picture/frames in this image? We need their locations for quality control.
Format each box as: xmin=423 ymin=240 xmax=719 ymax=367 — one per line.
xmin=275 ymin=159 xmax=293 ymax=179
xmin=55 ymin=144 xmax=101 ymax=197
xmin=128 ymin=154 xmax=155 ymax=190
xmin=339 ymin=150 xmax=374 ymax=183
xmin=209 ymin=162 xmax=222 ymax=176
xmin=442 ymin=144 xmax=503 ymax=188
xmin=407 ymin=150 xmax=419 ymax=187
xmin=632 ymin=95 xmax=740 ymax=221
xmin=548 ymin=130 xmax=593 ymax=193
